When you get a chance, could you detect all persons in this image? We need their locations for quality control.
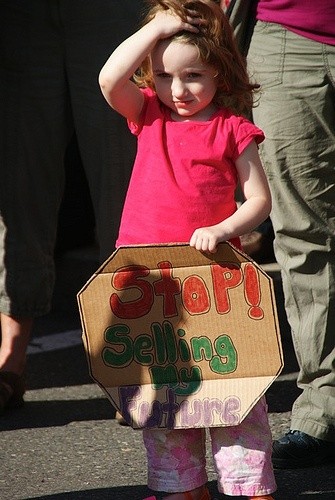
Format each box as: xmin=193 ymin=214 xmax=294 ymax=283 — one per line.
xmin=0 ymin=0 xmax=335 ymax=500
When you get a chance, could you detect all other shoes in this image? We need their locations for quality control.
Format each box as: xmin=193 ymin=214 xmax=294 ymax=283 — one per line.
xmin=140 ymin=486 xmax=212 ymax=500
xmin=0 ymin=369 xmax=28 ymax=418
xmin=249 ymin=495 xmax=276 ymax=500
xmin=271 ymin=430 xmax=335 ymax=469
